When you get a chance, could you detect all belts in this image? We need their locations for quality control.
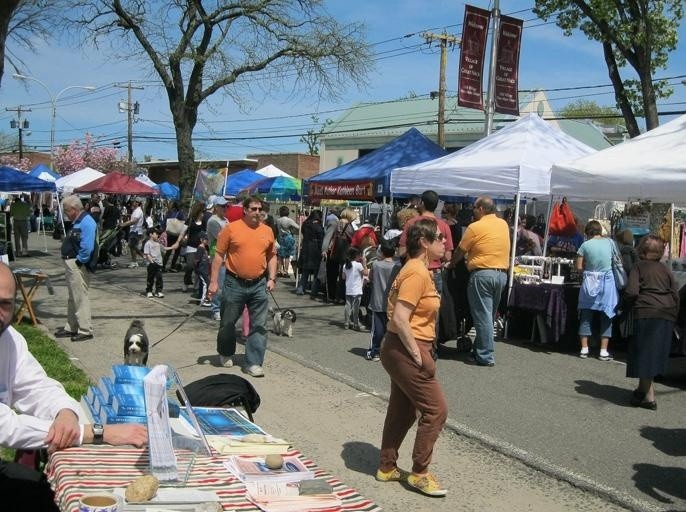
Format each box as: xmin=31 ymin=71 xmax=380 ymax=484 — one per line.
xmin=226 ymin=270 xmax=266 ymax=285
xmin=476 ymin=268 xmax=507 ymax=272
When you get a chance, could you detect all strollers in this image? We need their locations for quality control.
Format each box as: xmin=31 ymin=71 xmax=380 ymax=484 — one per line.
xmin=88 ymin=222 xmax=129 ymax=271
xmin=436 ymin=260 xmax=474 ymax=354
xmin=360 ymin=246 xmax=382 ymax=322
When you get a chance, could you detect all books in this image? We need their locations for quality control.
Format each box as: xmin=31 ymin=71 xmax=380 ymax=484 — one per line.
xmin=82 ymin=364 xmax=179 ymax=428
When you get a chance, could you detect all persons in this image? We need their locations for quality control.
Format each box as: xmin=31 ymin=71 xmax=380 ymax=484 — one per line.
xmin=0 ymin=261 xmax=151 ymax=512
xmin=376 ymin=217 xmax=448 ymax=497
xmin=0 ymin=190 xmax=680 ymax=410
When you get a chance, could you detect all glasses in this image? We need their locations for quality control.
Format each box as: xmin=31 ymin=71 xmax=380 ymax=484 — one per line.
xmin=249 ymin=208 xmax=262 ymax=211
xmin=435 ymin=233 xmax=443 ymax=240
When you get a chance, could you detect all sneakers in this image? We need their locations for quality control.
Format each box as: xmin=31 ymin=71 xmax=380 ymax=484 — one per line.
xmin=367 ymin=351 xmax=380 ymax=361
xmin=376 ymin=467 xmax=411 ymax=481
xmin=465 ymin=356 xmax=494 ymax=366
xmin=182 ymin=284 xmax=221 ymax=321
xmin=598 ymin=351 xmax=615 ymax=360
xmin=407 ymin=472 xmax=447 ymax=495
xmin=244 ymin=364 xmax=264 ymax=376
xmin=147 ymin=292 xmax=164 ymax=298
xmin=219 ymin=354 xmax=233 ymax=367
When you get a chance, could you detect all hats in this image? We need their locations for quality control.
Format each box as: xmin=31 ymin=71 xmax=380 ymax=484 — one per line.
xmin=205 ymin=195 xmax=232 ymax=209
xmin=130 ymin=197 xmax=142 ymax=202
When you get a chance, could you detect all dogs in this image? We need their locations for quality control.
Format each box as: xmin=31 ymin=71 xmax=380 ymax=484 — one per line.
xmin=268 ymin=306 xmax=296 ymax=338
xmin=123 ymin=319 xmax=149 ymax=368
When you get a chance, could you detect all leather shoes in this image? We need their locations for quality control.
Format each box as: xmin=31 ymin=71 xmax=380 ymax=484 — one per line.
xmin=630 ymin=389 xmax=657 ymax=410
xmin=54 ymin=330 xmax=93 ymax=341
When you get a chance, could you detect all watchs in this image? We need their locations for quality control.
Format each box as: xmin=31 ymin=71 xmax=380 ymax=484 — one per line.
xmin=93 ymin=423 xmax=104 ymax=445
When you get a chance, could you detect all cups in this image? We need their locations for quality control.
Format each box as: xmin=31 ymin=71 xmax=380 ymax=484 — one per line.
xmin=79 ymin=491 xmax=119 ymax=511
xmin=515 ymin=263 xmax=544 ymax=284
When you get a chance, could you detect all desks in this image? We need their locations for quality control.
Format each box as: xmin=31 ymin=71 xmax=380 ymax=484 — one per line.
xmin=14 ymin=268 xmax=50 ymax=328
xmin=509 ymin=273 xmax=581 ymax=347
xmin=39 ymin=403 xmax=384 ymax=510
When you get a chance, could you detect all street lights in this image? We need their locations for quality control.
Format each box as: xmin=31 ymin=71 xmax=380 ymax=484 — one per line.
xmin=18 ymin=129 xmax=32 ymax=163
xmin=12 ymin=74 xmax=98 ymax=174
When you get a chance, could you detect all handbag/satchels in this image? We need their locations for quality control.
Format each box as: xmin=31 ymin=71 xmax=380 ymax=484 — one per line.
xmin=612 ymin=254 xmax=628 ymax=290
xmin=166 ymin=218 xmax=185 ymax=236
xmin=334 ymin=238 xmax=352 ymax=263
xmin=532 ymin=202 xmax=611 ymax=239
